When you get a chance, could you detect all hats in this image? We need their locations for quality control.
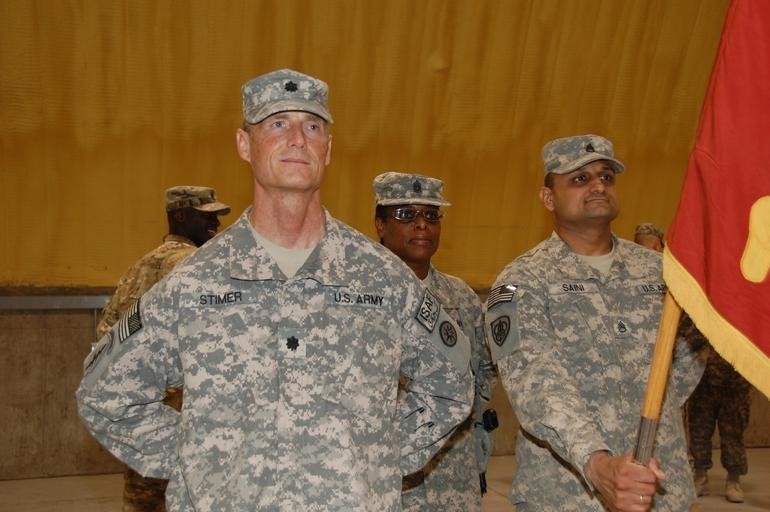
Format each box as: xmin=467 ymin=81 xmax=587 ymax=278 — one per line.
xmin=165 ymin=185 xmax=230 ymax=214
xmin=635 ymin=223 xmax=665 ymax=247
xmin=542 ymin=134 xmax=625 ymax=174
xmin=240 ymin=68 xmax=334 ymax=125
xmin=371 ymin=171 xmax=452 ymax=208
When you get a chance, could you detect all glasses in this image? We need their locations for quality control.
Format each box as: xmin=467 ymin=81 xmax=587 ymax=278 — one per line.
xmin=392 ymin=208 xmax=445 ymax=222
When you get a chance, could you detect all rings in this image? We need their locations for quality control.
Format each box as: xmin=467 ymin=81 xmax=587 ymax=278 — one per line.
xmin=639 ymin=494 xmax=645 ymax=505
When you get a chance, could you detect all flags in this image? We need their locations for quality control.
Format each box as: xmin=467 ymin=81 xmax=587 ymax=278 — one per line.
xmin=662 ymin=0 xmax=769 ymax=401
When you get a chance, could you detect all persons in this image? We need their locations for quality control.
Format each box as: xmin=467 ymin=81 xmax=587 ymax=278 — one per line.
xmin=686 ymin=320 xmax=750 ymax=502
xmin=75 ymin=69 xmax=476 ymax=512
xmin=634 ymin=222 xmax=695 ymax=472
xmin=96 ymin=185 xmax=233 ymax=511
xmin=372 ymin=175 xmax=497 ymax=512
xmin=483 ymin=134 xmax=699 ymax=511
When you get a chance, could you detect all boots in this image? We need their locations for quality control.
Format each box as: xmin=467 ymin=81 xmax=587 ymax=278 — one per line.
xmin=692 ymin=469 xmax=710 ymax=496
xmin=724 ymin=472 xmax=745 ymax=502
